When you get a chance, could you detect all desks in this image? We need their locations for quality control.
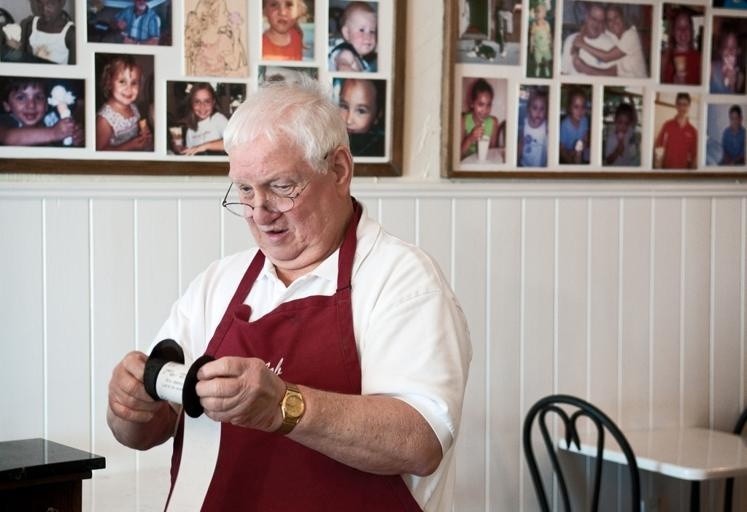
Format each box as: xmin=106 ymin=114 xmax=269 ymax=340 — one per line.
xmin=558 ymin=418 xmax=747 ymax=511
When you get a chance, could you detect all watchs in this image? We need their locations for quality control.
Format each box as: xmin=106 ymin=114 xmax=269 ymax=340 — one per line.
xmin=272 ymin=381 xmax=307 ymax=440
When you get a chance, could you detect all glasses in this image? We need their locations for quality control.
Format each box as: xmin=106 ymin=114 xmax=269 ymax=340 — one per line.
xmin=222 ymin=153 xmax=330 ymax=219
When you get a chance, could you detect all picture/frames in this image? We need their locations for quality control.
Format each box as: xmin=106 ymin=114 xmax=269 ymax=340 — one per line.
xmin=441 ymin=0 xmax=747 ymax=176
xmin=0 ymin=0 xmax=407 ymax=177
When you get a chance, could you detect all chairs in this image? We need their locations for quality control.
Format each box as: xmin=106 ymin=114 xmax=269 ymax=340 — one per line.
xmin=721 ymin=406 xmax=747 ymax=511
xmin=518 ymin=392 xmax=645 ymax=509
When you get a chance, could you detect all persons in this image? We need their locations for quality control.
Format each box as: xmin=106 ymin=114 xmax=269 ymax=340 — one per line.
xmin=107 ymin=74 xmax=473 ymax=511
xmin=719 ymin=103 xmax=746 ymax=166
xmin=561 ymin=89 xmax=591 ymax=160
xmin=662 ymin=7 xmax=702 ymax=87
xmin=337 ymin=79 xmax=386 ymax=133
xmin=531 ymin=5 xmax=553 ymax=79
xmin=327 ymin=2 xmax=379 ymax=74
xmin=172 ymin=81 xmax=231 ymax=152
xmin=1 ymin=0 xmax=78 ymax=65
xmin=99 ymin=54 xmax=151 ymax=152
xmin=712 ymin=19 xmax=743 ymax=91
xmin=460 ymin=80 xmax=499 ymax=159
xmin=264 ymin=0 xmax=311 ymax=64
xmin=561 ymin=3 xmax=648 ymax=75
xmin=471 ymin=0 xmax=518 ymax=62
xmin=654 ymin=91 xmax=698 ymax=166
xmin=113 ymin=0 xmax=162 ymax=45
xmin=605 ymin=103 xmax=635 ymax=166
xmin=518 ymin=95 xmax=548 ymax=166
xmin=1 ymin=79 xmax=87 ymax=147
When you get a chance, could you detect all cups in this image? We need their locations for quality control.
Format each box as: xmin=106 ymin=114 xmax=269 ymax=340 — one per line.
xmin=476 ymin=133 xmax=491 ymax=160
xmin=675 ymin=54 xmax=689 ymax=78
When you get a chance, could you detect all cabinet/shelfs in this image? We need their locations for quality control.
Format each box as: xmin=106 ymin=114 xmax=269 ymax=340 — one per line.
xmin=1 ymin=438 xmax=105 ymax=512
